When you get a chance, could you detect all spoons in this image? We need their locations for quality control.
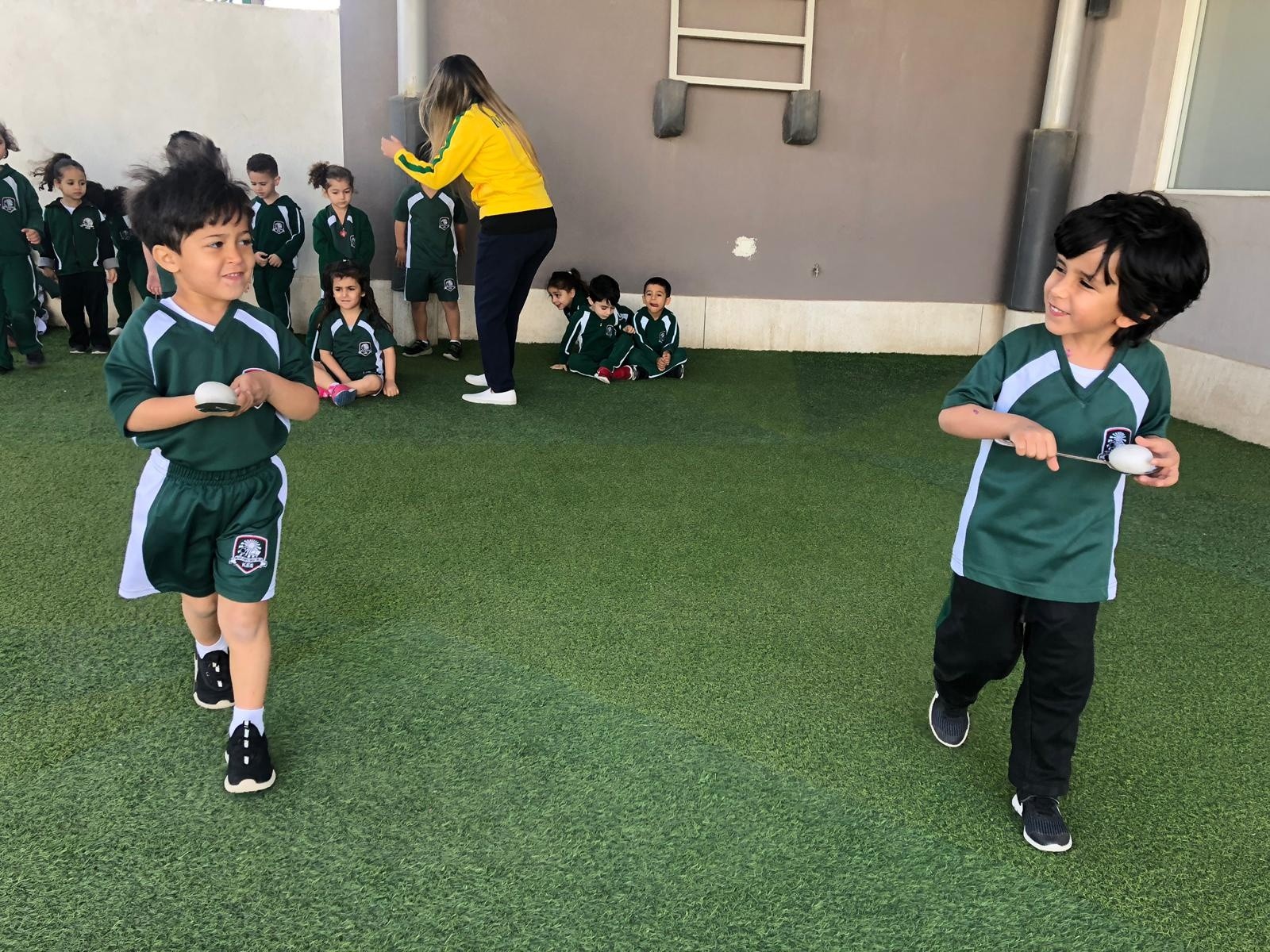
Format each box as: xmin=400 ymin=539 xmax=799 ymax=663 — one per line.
xmin=196 ymin=401 xmax=242 ymax=412
xmin=996 ymin=436 xmax=1165 ymax=474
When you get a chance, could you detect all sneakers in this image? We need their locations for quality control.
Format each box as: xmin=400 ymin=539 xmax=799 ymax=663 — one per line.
xmin=402 ymin=339 xmax=433 ymax=357
xmin=1010 ymin=791 xmax=1074 ymax=853
xmin=930 ymin=692 xmax=971 ymax=747
xmin=223 ymin=719 xmax=275 ymax=795
xmin=442 ymin=339 xmax=464 ymax=362
xmin=191 ymin=646 xmax=234 ymax=710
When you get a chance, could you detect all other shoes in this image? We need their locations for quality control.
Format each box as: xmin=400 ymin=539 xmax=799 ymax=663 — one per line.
xmin=92 ymin=343 xmax=111 ymax=354
xmin=109 ymin=327 xmax=123 ymax=336
xmin=631 ymin=364 xmax=650 ymax=381
xmin=664 ymin=364 xmax=686 ymax=378
xmin=612 ymin=367 xmax=630 ymax=380
xmin=70 ymin=346 xmax=89 ymax=354
xmin=317 ymin=386 xmax=329 ymax=398
xmin=462 ymin=386 xmax=517 ymax=406
xmin=595 ymin=368 xmax=612 ymax=384
xmin=0 ymin=365 xmax=14 ymax=375
xmin=8 ymin=337 xmax=17 ymax=348
xmin=465 ymin=373 xmax=489 ymax=387
xmin=27 ymin=350 xmax=46 ymax=365
xmin=330 ymin=384 xmax=357 ymax=406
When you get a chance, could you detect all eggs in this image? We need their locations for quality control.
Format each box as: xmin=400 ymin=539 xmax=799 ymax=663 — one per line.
xmin=194 ymin=381 xmax=238 ymax=404
xmin=1109 ymin=444 xmax=1154 ymax=473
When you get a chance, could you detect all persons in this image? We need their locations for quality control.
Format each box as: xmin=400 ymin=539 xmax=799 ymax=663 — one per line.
xmin=380 ymin=56 xmax=557 ymax=406
xmin=105 ymin=157 xmax=318 ymax=791
xmin=927 ymin=191 xmax=1211 ymax=855
xmin=307 ymin=261 xmax=399 ymax=407
xmin=0 ymin=123 xmax=178 ymax=376
xmin=395 ymin=140 xmax=467 ymax=360
xmin=548 ymin=268 xmax=688 ymax=385
xmin=246 ymin=153 xmax=305 ymax=333
xmin=308 ymin=162 xmax=375 ymax=298
